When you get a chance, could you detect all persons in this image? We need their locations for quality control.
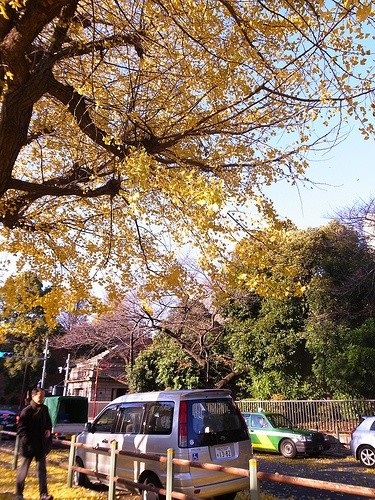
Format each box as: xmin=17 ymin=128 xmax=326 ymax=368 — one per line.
xmin=16 ymin=388 xmax=53 ymax=500
xmin=256 ymin=418 xmax=268 ymax=428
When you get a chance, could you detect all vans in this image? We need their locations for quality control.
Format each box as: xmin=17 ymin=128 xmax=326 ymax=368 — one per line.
xmin=74 ymin=390 xmax=253 ymax=500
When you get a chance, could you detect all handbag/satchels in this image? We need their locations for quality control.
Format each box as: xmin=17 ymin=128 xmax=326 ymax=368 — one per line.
xmin=42 ymin=433 xmax=52 ymax=455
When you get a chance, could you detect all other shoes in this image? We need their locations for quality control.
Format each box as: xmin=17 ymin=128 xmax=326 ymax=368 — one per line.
xmin=13 ymin=494 xmax=23 ymax=500
xmin=40 ymin=493 xmax=53 ymax=500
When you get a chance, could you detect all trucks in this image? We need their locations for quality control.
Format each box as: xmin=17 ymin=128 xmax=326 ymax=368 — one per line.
xmin=42 ymin=396 xmax=89 ymax=440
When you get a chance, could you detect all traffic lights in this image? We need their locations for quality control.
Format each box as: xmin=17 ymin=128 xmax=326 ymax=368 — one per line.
xmin=0 ymin=352 xmax=13 ymax=358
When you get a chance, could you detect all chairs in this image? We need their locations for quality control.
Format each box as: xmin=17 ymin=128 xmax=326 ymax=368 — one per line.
xmin=150 ymin=410 xmax=172 ymax=435
xmin=126 ymin=424 xmax=145 ymax=433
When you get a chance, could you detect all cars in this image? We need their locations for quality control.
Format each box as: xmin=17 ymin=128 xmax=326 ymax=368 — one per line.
xmin=0 ymin=410 xmax=20 ymax=432
xmin=241 ymin=408 xmax=325 ymax=459
xmin=350 ymin=416 xmax=375 ymax=468
